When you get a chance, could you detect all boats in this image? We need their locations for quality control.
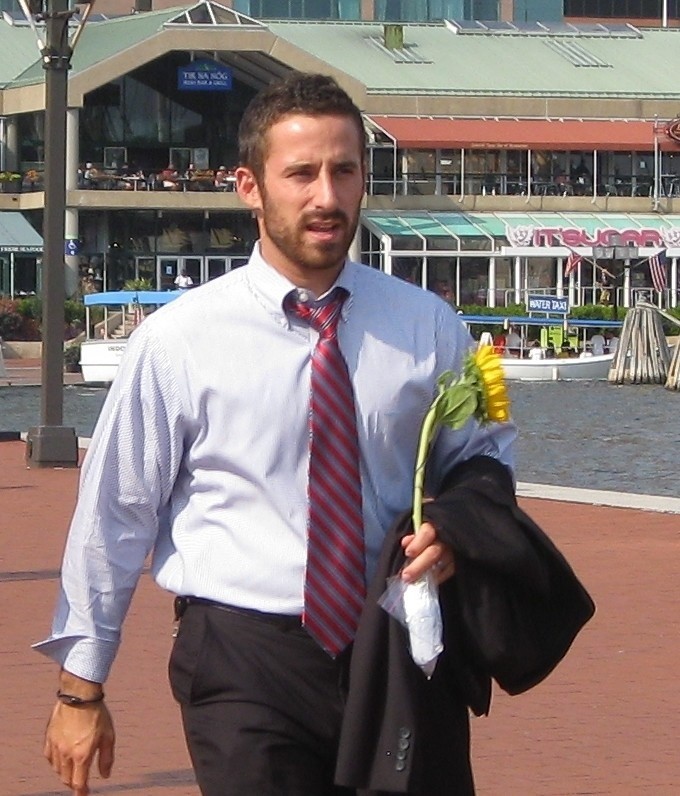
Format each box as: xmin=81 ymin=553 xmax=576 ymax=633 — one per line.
xmin=459 ymin=314 xmax=675 ymax=383
xmin=78 ymin=289 xmax=189 ymax=389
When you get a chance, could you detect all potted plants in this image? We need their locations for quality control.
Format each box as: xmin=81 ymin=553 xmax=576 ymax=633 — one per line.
xmin=121 ymin=276 xmax=158 ymax=315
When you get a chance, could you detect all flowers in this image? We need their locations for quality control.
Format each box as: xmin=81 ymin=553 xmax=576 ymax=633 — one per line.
xmin=412 ymin=342 xmax=512 ymax=534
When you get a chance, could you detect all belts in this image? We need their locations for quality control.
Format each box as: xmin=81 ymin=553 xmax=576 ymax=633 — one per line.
xmin=184 ymin=597 xmax=306 ymax=635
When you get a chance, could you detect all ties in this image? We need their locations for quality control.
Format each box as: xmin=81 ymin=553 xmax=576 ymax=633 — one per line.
xmin=283 ymin=290 xmax=368 ymax=660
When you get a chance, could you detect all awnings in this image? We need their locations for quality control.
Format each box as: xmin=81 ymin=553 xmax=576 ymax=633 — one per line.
xmin=0 ymin=212 xmax=43 ymax=252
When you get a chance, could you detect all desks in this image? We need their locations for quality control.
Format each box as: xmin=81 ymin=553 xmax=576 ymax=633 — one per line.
xmin=176 ymin=177 xmax=189 ymax=192
xmin=223 ymin=177 xmax=236 ymax=192
xmin=88 ymin=174 xmax=146 ymax=191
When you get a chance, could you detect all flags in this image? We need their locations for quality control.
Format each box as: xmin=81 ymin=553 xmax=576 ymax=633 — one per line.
xmin=563 ymin=244 xmax=581 ymax=277
xmin=650 ymin=250 xmax=667 ymax=293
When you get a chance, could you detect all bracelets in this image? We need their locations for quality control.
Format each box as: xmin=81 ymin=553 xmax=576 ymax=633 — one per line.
xmin=56 ymin=690 xmax=104 ymax=706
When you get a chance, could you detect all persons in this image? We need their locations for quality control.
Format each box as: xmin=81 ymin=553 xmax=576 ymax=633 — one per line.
xmin=31 ymin=73 xmax=519 ymax=796
xmin=162 ymin=163 xmax=229 ymax=191
xmin=556 ymin=171 xmax=567 ymax=197
xmin=85 ymin=162 xmax=145 ymax=189
xmin=604 ymin=329 xmax=619 ymax=354
xmin=494 ymin=325 xmax=609 ymax=360
xmin=174 ymin=269 xmax=193 ymax=290
